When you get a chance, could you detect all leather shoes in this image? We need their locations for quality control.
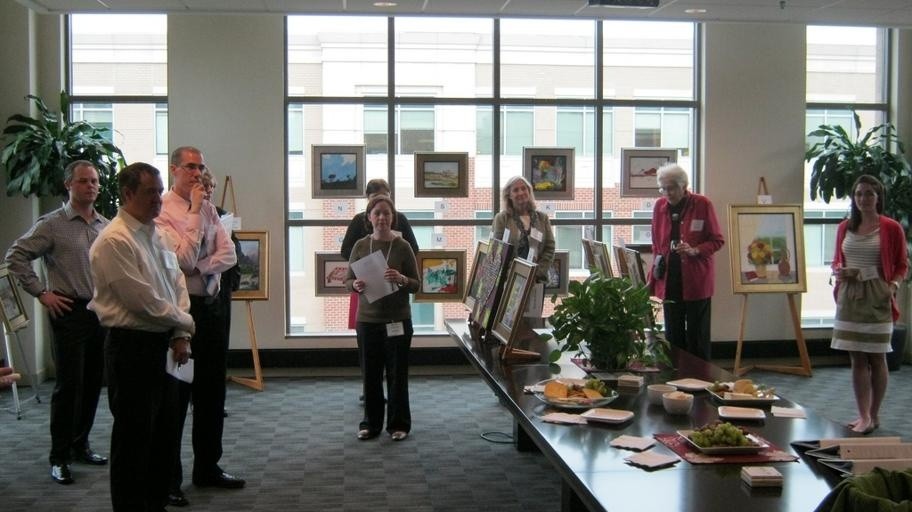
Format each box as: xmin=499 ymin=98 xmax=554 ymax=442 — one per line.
xmin=51 ymin=465 xmax=73 ymax=484
xmin=357 ymin=428 xmax=377 ymax=440
xmin=847 ymin=421 xmax=879 ymax=434
xmin=76 ymin=448 xmax=108 ymax=465
xmin=392 ymin=431 xmax=407 ymax=441
xmin=213 ymin=471 xmax=246 ymax=488
xmin=169 ymin=489 xmax=189 ymax=507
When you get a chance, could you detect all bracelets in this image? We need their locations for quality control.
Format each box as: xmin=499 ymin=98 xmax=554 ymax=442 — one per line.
xmin=39 ymin=290 xmax=47 ymax=299
xmin=891 ymin=280 xmax=901 ymax=293
xmin=397 ymin=274 xmax=406 ymax=287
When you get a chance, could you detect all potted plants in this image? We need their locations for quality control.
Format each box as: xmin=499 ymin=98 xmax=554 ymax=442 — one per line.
xmin=544 ymin=271 xmax=674 ymax=375
xmin=802 ymin=105 xmax=911 ymax=371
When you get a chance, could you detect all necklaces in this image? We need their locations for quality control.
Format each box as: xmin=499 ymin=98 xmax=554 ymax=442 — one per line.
xmin=368 ymin=232 xmax=394 ymax=265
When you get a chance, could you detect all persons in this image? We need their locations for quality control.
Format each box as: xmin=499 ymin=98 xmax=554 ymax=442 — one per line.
xmin=493 ymin=176 xmax=556 ymax=303
xmin=831 ymin=175 xmax=910 ymax=435
xmin=647 ymin=164 xmax=725 ymax=361
xmin=341 ymin=178 xmax=418 ymax=402
xmin=5 ymin=160 xmax=112 ymax=486
xmin=158 ymin=146 xmax=246 ymax=508
xmin=203 ymin=169 xmax=241 ymax=417
xmin=344 ymin=194 xmax=422 ymax=440
xmin=86 ymin=163 xmax=195 ymax=511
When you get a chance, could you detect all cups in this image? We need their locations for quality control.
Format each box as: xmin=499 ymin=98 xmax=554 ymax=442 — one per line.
xmin=670 ymin=240 xmax=683 ymax=256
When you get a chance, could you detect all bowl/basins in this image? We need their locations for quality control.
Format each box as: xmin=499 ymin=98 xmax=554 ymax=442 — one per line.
xmin=662 ymin=392 xmax=695 ymax=414
xmin=839 ymin=268 xmax=861 ymax=278
xmin=647 ymin=384 xmax=677 ymax=405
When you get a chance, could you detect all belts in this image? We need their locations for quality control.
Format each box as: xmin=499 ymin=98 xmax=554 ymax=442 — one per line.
xmin=190 ymin=295 xmax=216 ymax=305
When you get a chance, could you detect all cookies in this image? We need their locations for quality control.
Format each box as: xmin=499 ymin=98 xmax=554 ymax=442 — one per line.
xmin=544 ymin=383 xmax=603 ymax=399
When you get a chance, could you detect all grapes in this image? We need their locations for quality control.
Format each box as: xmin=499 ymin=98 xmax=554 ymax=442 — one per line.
xmin=584 ymin=378 xmax=607 ymax=394
xmin=688 ymin=420 xmax=751 ymax=448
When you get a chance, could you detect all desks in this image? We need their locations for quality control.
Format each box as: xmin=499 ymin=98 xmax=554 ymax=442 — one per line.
xmin=446 ymin=319 xmax=912 ymax=512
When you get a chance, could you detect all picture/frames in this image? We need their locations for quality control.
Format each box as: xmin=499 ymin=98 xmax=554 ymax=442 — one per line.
xmin=314 ymin=251 xmax=351 ymax=295
xmin=620 ymin=147 xmax=678 ymax=197
xmin=548 ymin=249 xmax=570 ymax=291
xmin=522 ymin=147 xmax=575 ymax=202
xmin=582 ymin=238 xmax=655 ymax=334
xmin=413 ymin=248 xmax=468 ymax=302
xmin=226 ymin=230 xmax=271 ymax=302
xmin=310 ymin=144 xmax=366 ymax=199
xmin=463 ymin=240 xmax=541 ymax=362
xmin=0 ymin=263 xmax=30 ymax=334
xmin=412 ymin=150 xmax=469 ymax=198
xmin=727 ymin=201 xmax=807 ymax=296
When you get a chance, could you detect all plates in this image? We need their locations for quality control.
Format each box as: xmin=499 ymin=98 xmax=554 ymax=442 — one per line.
xmin=533 ymin=378 xmax=619 ymax=408
xmin=676 ymin=430 xmax=770 ymax=454
xmin=704 ymin=384 xmax=781 ymax=405
